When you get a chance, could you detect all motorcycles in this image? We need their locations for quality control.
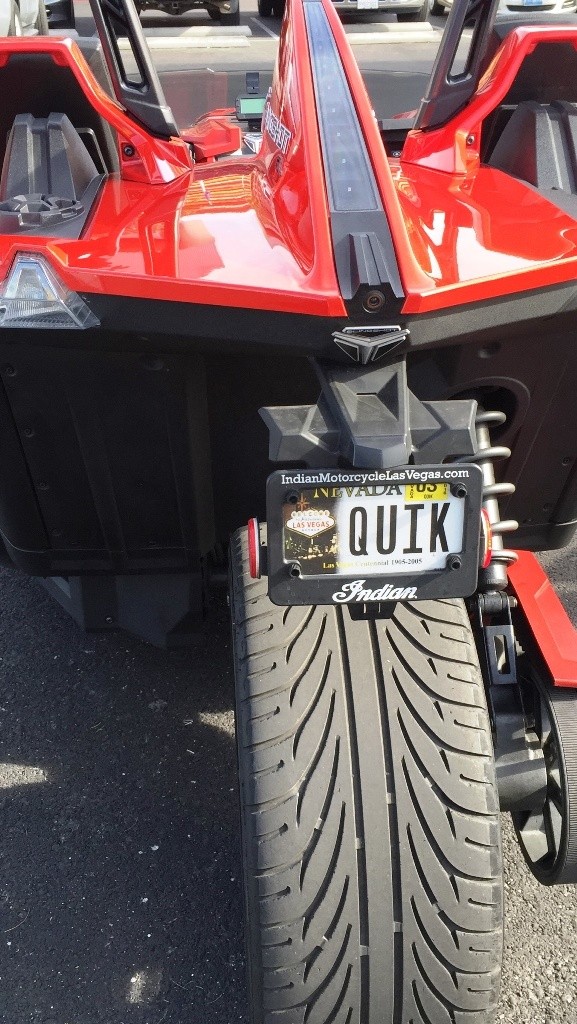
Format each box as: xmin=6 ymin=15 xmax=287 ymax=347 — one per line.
xmin=0 ymin=0 xmax=577 ymax=1024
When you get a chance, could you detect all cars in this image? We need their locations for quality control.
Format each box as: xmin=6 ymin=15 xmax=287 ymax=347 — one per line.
xmin=15 ymin=0 xmax=577 ymax=34
